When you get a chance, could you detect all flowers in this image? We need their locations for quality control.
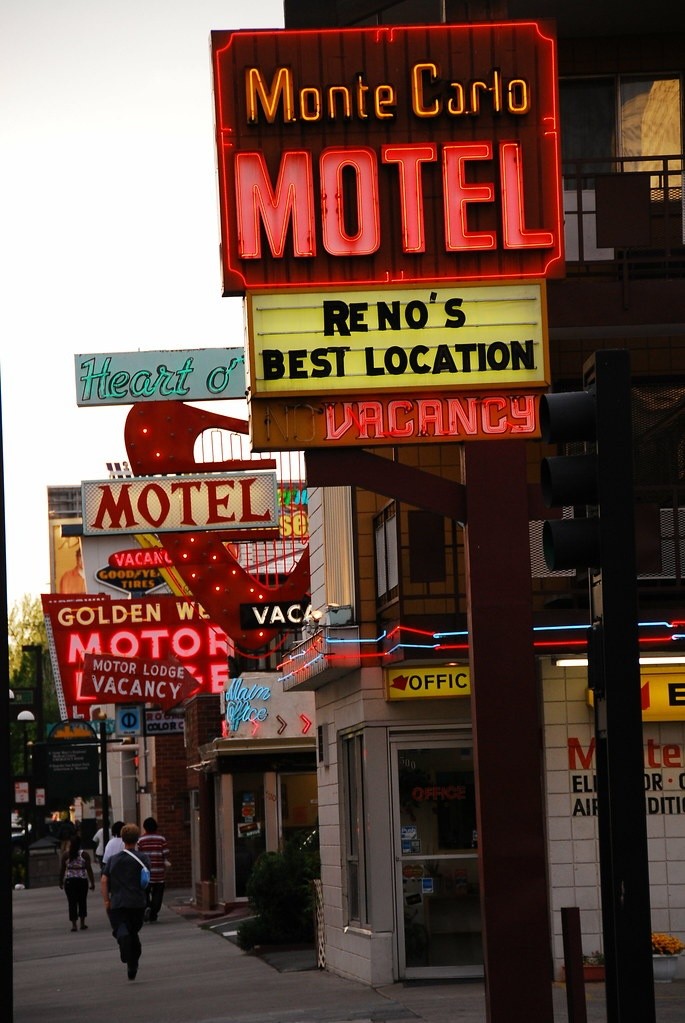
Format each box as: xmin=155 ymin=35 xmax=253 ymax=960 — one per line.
xmin=583 ymin=951 xmax=605 ymax=965
xmin=651 ymin=931 xmax=685 ymax=956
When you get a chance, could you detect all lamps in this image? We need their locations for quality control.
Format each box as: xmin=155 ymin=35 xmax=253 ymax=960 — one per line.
xmin=311 ymin=611 xmax=323 ymax=633
xmin=549 ymin=651 xmax=685 ymax=668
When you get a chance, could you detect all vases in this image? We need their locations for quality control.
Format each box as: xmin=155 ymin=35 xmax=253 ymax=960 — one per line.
xmin=562 ymin=965 xmax=605 ymax=983
xmin=652 ymin=954 xmax=680 ymax=984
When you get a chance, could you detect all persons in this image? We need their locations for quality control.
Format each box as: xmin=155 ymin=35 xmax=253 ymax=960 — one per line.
xmin=60 ymin=819 xmax=80 ymax=850
xmin=93 ymin=820 xmax=113 ymax=866
xmin=137 ymin=817 xmax=169 ymax=922
xmin=102 ymin=821 xmax=125 ymax=863
xmin=59 ymin=835 xmax=95 ymax=931
xmin=100 ymin=823 xmax=151 ymax=980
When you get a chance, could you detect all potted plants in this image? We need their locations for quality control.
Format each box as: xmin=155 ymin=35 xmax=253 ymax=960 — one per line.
xmin=421 ymin=860 xmax=442 ymax=895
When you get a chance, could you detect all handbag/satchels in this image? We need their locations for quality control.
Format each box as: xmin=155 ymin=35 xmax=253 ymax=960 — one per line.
xmin=164 ymin=858 xmax=175 ymax=881
xmin=140 ymin=867 xmax=151 ymax=888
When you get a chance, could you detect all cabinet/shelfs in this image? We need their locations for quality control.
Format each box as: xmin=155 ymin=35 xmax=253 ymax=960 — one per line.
xmin=424 ymin=894 xmax=481 ymax=967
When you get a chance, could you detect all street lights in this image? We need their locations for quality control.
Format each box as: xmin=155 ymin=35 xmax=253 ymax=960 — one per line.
xmin=17 ymin=710 xmax=37 ymax=847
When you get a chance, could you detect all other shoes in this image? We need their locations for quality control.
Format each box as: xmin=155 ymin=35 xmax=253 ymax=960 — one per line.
xmin=143 ymin=906 xmax=152 ymax=922
xmin=80 ymin=925 xmax=88 ymax=929
xmin=71 ymin=928 xmax=77 ymax=931
xmin=127 ymin=954 xmax=138 ymax=980
xmin=120 ymin=935 xmax=131 ymax=963
xmin=149 ymin=918 xmax=156 ymax=923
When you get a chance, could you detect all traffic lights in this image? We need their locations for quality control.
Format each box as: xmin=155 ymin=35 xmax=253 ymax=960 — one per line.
xmin=534 ymin=344 xmax=633 ymax=576
